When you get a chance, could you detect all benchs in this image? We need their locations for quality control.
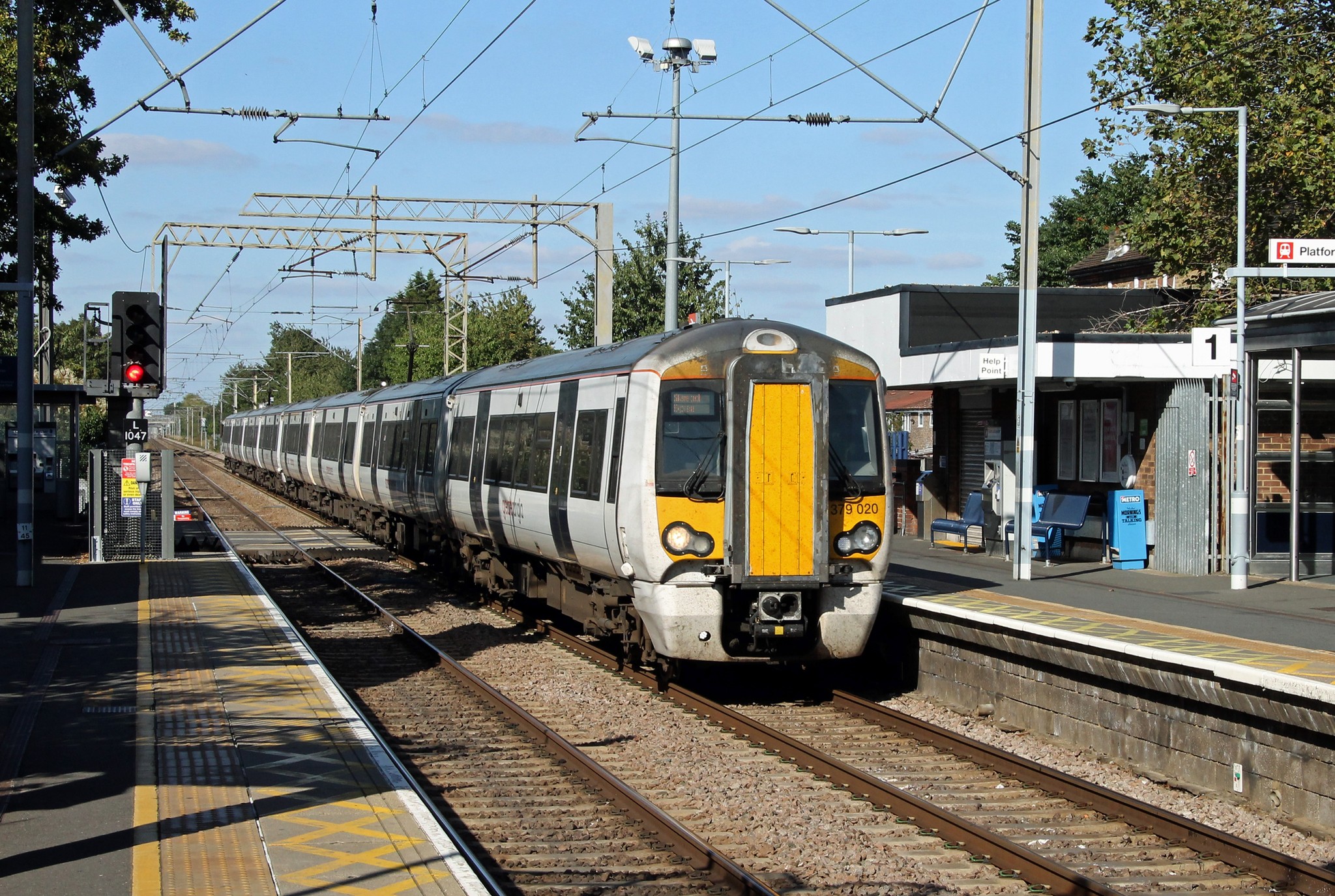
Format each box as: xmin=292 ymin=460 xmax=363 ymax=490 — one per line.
xmin=929 ymin=491 xmax=983 ymax=555
xmin=1002 ymin=489 xmax=1093 ymax=568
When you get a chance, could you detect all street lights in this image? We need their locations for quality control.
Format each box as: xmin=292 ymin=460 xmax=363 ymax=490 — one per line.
xmin=630 ymin=36 xmax=718 ymax=333
xmin=773 ymin=226 xmax=929 ymax=296
xmin=663 ymin=257 xmax=792 ymax=320
xmin=1122 ymin=104 xmax=1250 ymax=590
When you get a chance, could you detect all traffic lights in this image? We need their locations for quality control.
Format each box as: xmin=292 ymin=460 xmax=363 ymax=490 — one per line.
xmin=112 ymin=291 xmax=161 ymax=387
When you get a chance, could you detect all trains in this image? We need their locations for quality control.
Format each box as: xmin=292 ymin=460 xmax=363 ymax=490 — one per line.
xmin=222 ymin=319 xmax=895 ymax=671
